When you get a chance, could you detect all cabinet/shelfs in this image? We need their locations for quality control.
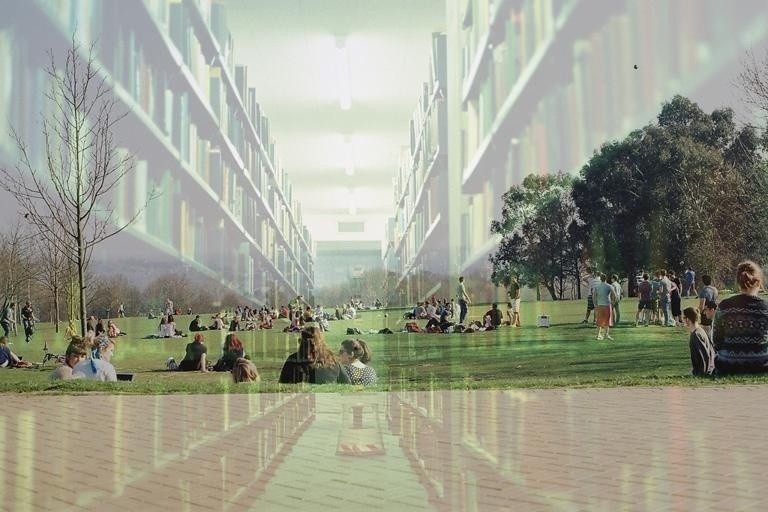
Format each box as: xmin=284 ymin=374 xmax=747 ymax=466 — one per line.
xmin=388 ymin=0 xmax=766 ymax=511
xmin=1 ymin=0 xmax=321 ymax=511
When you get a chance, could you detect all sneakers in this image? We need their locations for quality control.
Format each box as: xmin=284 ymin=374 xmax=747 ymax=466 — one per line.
xmin=597 ymin=333 xmax=616 ymax=342
xmin=580 ymin=316 xmax=684 ymax=328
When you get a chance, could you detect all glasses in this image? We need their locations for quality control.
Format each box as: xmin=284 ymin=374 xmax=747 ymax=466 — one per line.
xmin=339 ymin=349 xmax=348 ymax=354
